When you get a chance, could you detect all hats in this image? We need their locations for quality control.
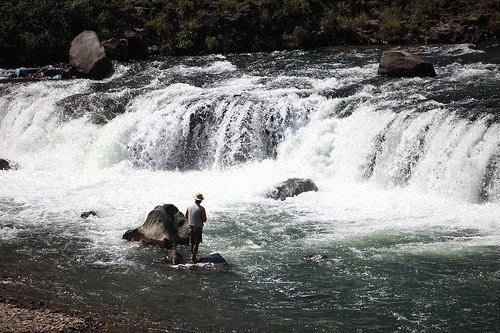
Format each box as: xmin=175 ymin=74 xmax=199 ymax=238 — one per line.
xmin=194 ymin=193 xmax=205 ymax=201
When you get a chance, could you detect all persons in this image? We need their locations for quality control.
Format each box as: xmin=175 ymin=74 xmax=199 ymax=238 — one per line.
xmin=185 ymin=193 xmax=207 ymax=264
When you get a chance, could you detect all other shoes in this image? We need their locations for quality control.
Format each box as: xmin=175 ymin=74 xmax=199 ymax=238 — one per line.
xmin=190 ymin=256 xmax=201 ymax=261
